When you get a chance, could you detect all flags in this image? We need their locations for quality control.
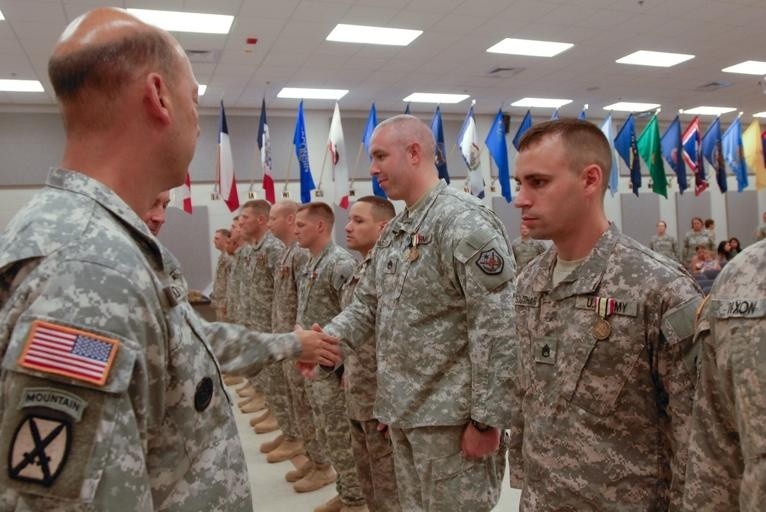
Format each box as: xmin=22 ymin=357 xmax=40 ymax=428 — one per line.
xmin=405 ymin=101 xmax=532 ymax=203
xmin=552 ymin=109 xmax=765 ymax=199
xmin=216 ymin=97 xmax=386 ymax=213
xmin=166 ymin=174 xmax=192 ymax=216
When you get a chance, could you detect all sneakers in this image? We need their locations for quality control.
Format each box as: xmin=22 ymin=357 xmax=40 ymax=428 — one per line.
xmin=235 ymin=382 xmax=254 ymax=397
xmin=339 ymin=504 xmax=369 ymax=512
xmin=285 ymin=461 xmax=313 ymax=482
xmin=312 ymin=494 xmax=344 ymax=512
xmin=249 ymin=409 xmax=270 ymax=427
xmin=266 ymin=440 xmax=305 ymax=463
xmin=259 ymin=434 xmax=284 ymax=454
xmin=222 ymin=375 xmax=241 ymax=386
xmin=293 ymin=463 xmax=337 ymax=493
xmin=238 ymin=391 xmax=266 ymax=413
xmin=253 ymin=414 xmax=279 ymax=434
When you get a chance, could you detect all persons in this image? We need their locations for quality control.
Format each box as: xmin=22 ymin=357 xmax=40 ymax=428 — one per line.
xmin=142 ymin=190 xmax=170 ymax=236
xmin=511 ymin=221 xmax=548 ymax=277
xmin=292 ymin=114 xmax=516 ymax=512
xmin=209 ymin=195 xmax=402 ymax=511
xmin=0 ymin=6 xmax=252 ymax=512
xmin=681 ymin=236 xmax=766 ymax=512
xmin=645 ymin=212 xmax=766 ymax=274
xmin=505 ymin=116 xmax=706 ymax=512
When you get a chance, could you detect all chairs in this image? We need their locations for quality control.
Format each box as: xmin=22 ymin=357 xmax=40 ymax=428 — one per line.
xmin=691 ymin=261 xmax=727 ymax=298
